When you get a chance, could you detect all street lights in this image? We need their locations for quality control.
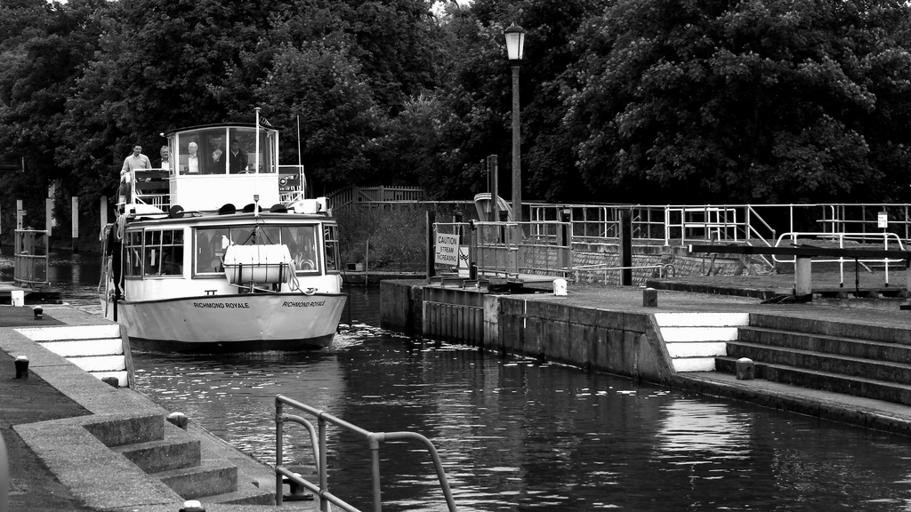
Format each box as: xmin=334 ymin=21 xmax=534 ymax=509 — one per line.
xmin=501 ymin=21 xmax=525 ymax=272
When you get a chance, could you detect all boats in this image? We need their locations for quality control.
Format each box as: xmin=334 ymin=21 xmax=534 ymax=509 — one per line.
xmin=94 ymin=98 xmax=349 ymax=357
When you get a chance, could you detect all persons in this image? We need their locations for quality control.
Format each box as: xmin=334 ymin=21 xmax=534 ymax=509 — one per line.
xmin=106 ymin=134 xmax=249 ymax=299
xmin=453 ymin=205 xmax=464 ymax=245
